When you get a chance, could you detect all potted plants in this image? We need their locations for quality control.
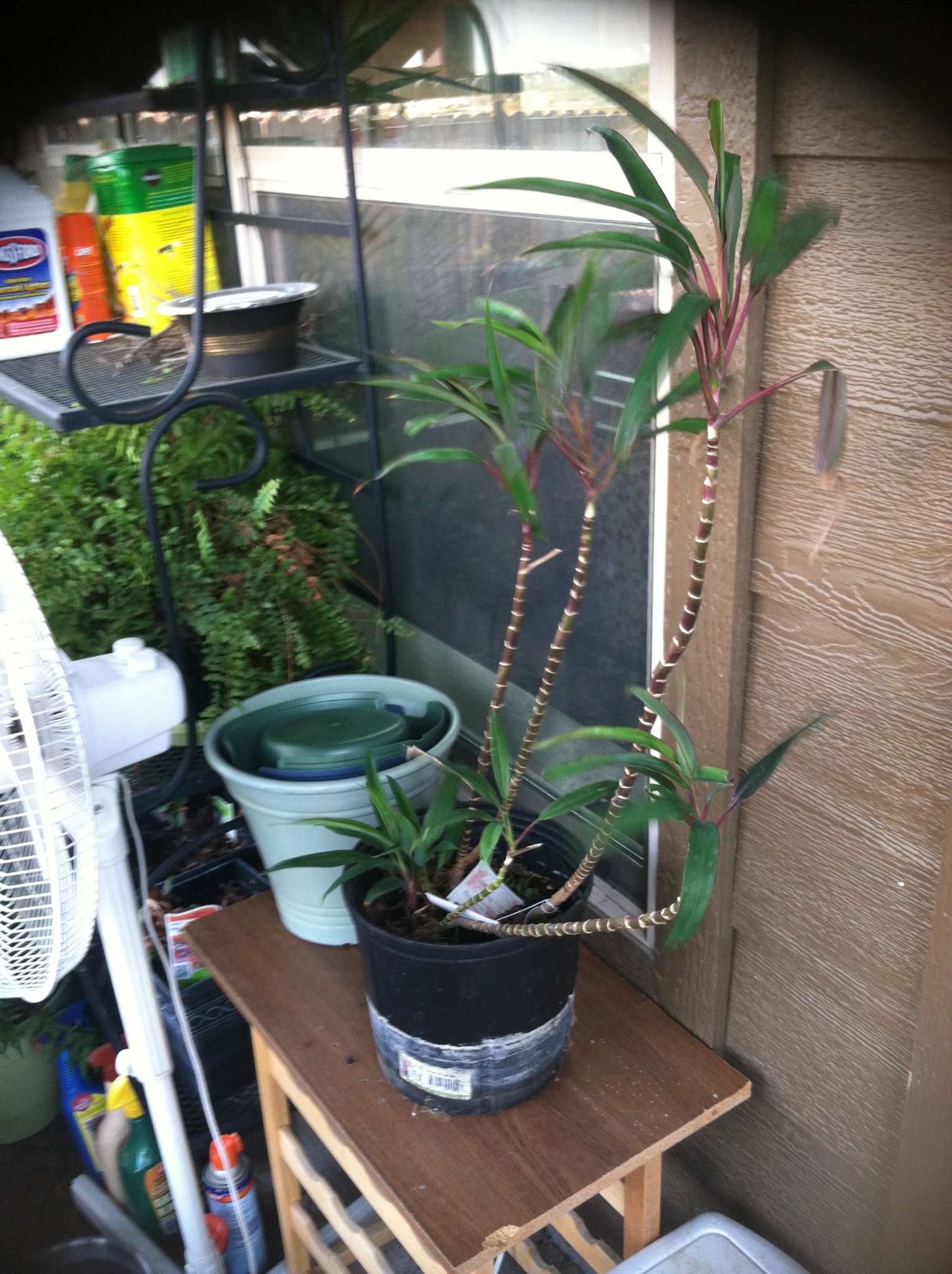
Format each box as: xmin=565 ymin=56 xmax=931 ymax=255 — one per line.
xmin=243 ymin=64 xmax=845 ymax=1117
xmin=1 ymin=976 xmax=105 ymax=1145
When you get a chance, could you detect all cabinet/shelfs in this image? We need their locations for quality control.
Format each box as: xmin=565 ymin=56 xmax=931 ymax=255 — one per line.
xmin=0 ymin=0 xmax=394 ymax=902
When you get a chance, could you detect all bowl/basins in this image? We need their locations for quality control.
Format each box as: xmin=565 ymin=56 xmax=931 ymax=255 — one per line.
xmin=157 ymin=282 xmax=322 ymax=380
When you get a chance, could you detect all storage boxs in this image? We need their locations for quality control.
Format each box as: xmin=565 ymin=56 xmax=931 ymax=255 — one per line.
xmin=76 ymin=859 xmax=270 ymax=1158
xmin=87 ymin=144 xmax=222 ymax=336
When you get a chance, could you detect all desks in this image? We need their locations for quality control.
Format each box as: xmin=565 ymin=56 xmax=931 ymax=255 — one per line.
xmin=183 ymin=890 xmax=752 ymax=1274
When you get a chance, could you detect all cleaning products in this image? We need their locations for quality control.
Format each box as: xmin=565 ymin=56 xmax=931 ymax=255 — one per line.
xmin=84 ymin=1031 xmax=132 ymax=1210
xmin=0 ymin=158 xmax=76 ymax=365
xmin=32 ymin=996 xmax=111 ymax=1179
xmin=200 ymin=1132 xmax=274 ymax=1274
xmin=104 ymin=1074 xmax=185 ymax=1256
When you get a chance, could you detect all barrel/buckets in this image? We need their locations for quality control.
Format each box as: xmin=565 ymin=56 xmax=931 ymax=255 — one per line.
xmin=69 ymin=145 xmax=223 ymax=338
xmin=204 ymin=673 xmax=462 ymax=947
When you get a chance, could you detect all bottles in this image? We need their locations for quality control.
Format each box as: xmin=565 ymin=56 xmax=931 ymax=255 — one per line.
xmin=56 ymin=181 xmax=114 ymax=344
xmin=0 ymin=160 xmax=75 ymax=362
xmin=56 ymin=1043 xmax=269 ymax=1274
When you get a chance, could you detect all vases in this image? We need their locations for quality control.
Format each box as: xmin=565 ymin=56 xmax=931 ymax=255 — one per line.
xmin=203 ymin=673 xmax=461 ymax=947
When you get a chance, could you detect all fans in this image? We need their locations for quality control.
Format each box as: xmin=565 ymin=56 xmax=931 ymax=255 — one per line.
xmin=0 ymin=532 xmax=221 ymax=1273
